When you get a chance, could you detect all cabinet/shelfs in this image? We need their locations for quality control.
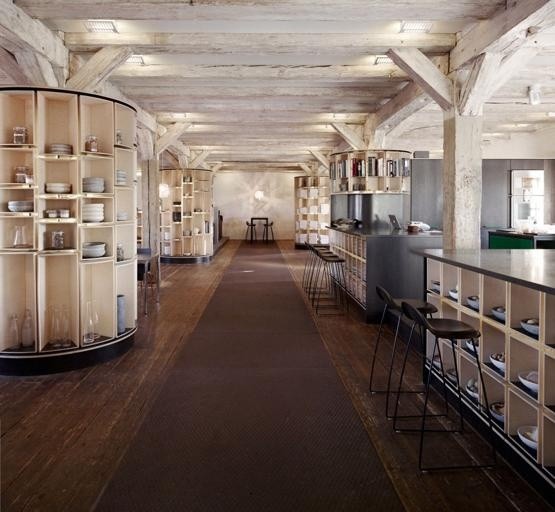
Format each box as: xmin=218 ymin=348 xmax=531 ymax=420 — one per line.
xmin=2 ymin=85 xmax=138 ymax=376
xmin=137 ymin=168 xmax=216 ymax=265
xmin=295 ymin=175 xmax=330 ymax=249
xmin=423 ymin=259 xmax=553 ymax=485
xmin=329 ymin=150 xmax=411 ymax=311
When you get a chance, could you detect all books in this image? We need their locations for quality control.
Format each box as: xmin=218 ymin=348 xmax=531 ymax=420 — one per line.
xmin=330 ymin=156 xmax=410 ymax=180
xmin=298 ymin=177 xmax=315 ymax=187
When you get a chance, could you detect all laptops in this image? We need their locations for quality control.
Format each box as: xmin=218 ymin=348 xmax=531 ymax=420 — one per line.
xmin=389 ymin=213 xmax=406 ymax=230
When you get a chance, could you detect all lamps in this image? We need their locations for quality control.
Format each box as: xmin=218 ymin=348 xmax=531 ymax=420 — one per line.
xmin=527 ymin=85 xmax=541 ymax=106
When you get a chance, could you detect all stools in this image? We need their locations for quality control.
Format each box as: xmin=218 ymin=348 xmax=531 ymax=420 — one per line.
xmin=392 ymin=299 xmax=495 ymax=472
xmin=302 ymin=240 xmax=348 ymax=316
xmin=369 ymin=285 xmax=451 ymax=420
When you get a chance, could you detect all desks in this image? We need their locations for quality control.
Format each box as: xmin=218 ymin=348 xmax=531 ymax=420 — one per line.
xmin=251 ymin=218 xmax=268 ymax=243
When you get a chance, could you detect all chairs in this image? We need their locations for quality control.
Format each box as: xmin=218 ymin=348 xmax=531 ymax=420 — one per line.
xmin=262 ymin=221 xmax=275 ymax=244
xmin=137 ymin=248 xmax=150 ymax=280
xmin=136 ymin=253 xmax=160 ymax=316
xmin=244 ymin=221 xmax=258 ymax=244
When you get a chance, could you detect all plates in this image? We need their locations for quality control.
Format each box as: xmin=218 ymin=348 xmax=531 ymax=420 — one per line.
xmin=116 ymin=169 xmax=129 ymax=221
xmin=6 ymin=201 xmax=34 ymax=213
xmin=47 ymin=142 xmax=74 ymax=154
xmin=45 ymin=182 xmax=72 ymax=193
xmin=82 ymin=202 xmax=105 ymax=223
xmin=82 ymin=176 xmax=105 ymax=193
xmin=430 ymin=278 xmax=540 ymax=452
xmin=81 ymin=242 xmax=106 ymax=257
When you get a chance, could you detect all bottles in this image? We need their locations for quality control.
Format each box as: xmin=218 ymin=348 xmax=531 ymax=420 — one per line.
xmin=21 ymin=309 xmax=33 ymax=346
xmin=9 ymin=225 xmax=31 ymax=248
xmin=115 ymin=127 xmax=126 ymax=144
xmin=117 ymin=242 xmax=125 ymax=263
xmin=14 ymin=165 xmax=26 ymax=183
xmin=49 ymin=306 xmax=62 ymax=348
xmin=46 ymin=210 xmax=57 ymax=217
xmin=85 ymin=133 xmax=98 ymax=152
xmin=61 ymin=306 xmax=73 ymax=346
xmin=58 ymin=209 xmax=69 ymax=217
xmin=92 ymin=300 xmax=99 ymax=339
xmin=13 ymin=127 xmax=26 ymax=144
xmin=5 ymin=312 xmax=21 ymax=349
xmin=83 ymin=302 xmax=94 ymax=344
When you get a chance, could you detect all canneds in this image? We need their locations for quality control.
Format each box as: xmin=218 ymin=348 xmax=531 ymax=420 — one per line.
xmin=85 ymin=135 xmax=98 ymax=152
xmin=13 ymin=127 xmax=28 ymax=144
xmin=117 ymin=244 xmax=124 ymax=261
